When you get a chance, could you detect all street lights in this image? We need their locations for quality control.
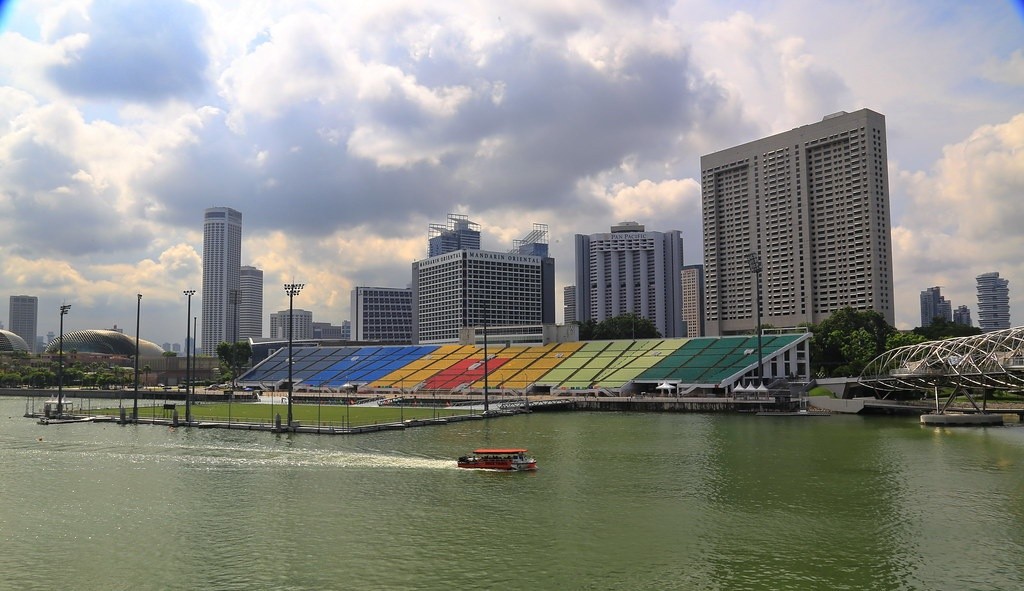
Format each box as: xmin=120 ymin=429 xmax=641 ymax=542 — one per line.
xmin=183 ymin=290 xmax=196 ymax=422
xmin=57 ymin=305 xmax=73 ymax=420
xmin=132 ymin=294 xmax=142 ymax=419
xmin=284 ymin=283 xmax=305 ymax=425
xmin=746 ymin=252 xmax=762 ymax=385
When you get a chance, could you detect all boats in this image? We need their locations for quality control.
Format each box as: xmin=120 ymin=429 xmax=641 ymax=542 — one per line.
xmin=458 ymin=448 xmax=537 ymax=471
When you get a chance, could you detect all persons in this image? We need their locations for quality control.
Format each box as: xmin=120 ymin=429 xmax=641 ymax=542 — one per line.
xmin=473 ymin=455 xmax=526 ymax=465
